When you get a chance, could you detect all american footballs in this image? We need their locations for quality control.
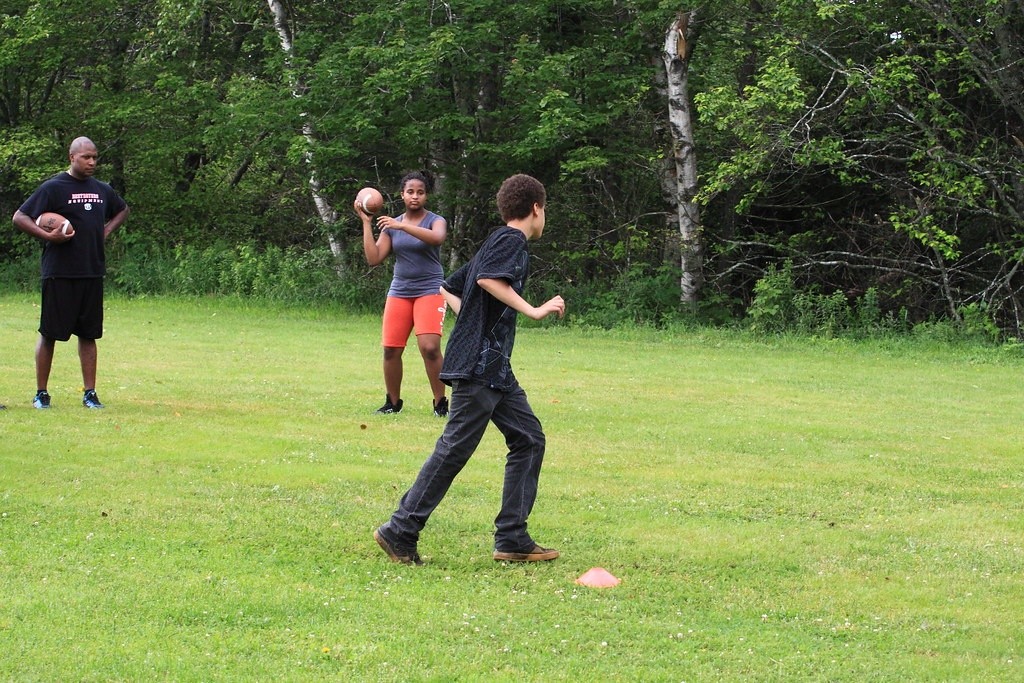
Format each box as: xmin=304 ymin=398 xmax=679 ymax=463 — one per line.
xmin=35 ymin=212 xmax=73 ymax=240
xmin=356 ymin=187 xmax=384 ymax=214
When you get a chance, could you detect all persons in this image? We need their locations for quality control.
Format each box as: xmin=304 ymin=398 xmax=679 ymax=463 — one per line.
xmin=373 ymin=173 xmax=565 ymax=567
xmin=13 ymin=137 xmax=130 ymax=410
xmin=354 ymin=172 xmax=449 ymax=418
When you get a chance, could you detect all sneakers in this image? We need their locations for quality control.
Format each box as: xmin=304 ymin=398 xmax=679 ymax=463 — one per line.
xmin=493 ymin=542 xmax=559 ymax=561
xmin=374 ymin=394 xmax=403 ymax=415
xmin=374 ymin=530 xmax=425 ymax=565
xmin=433 ymin=397 xmax=449 ymax=417
xmin=33 ymin=392 xmax=50 ymax=410
xmin=83 ymin=390 xmax=103 ymax=409
